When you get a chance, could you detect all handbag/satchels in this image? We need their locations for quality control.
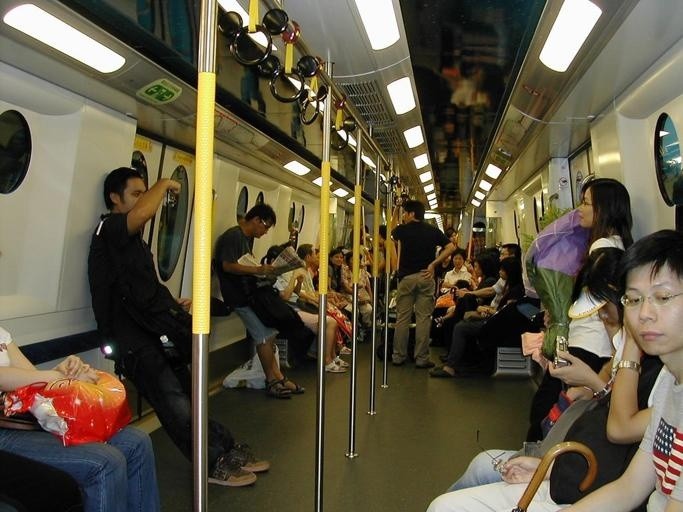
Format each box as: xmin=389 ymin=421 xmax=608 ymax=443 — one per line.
xmin=297 ymin=299 xmax=319 ymax=314
xmin=550 ymin=404 xmax=629 ymax=504
xmin=464 ymin=311 xmax=481 ymax=320
xmin=357 ymin=288 xmax=371 ymax=304
xmin=539 ymin=390 xmax=572 ymax=439
xmin=248 ymin=284 xmax=287 ymax=325
xmin=229 ymin=443 xmax=270 ymax=472
xmin=138 ymin=283 xmax=191 ymax=365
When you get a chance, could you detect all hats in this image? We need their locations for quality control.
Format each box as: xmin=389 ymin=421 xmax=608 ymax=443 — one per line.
xmin=568 ymin=285 xmax=615 ymax=318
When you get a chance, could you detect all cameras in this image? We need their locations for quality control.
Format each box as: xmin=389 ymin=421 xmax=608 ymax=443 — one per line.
xmin=164 ymin=189 xmax=177 ymax=208
xmin=553 ymin=335 xmax=569 ymax=369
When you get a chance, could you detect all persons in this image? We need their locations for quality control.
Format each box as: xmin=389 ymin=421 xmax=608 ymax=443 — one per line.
xmin=526 ymin=177 xmax=634 ymax=441
xmin=215 ymin=202 xmax=308 ymax=400
xmin=87 ymin=164 xmax=270 ymax=488
xmin=429 ymin=228 xmax=528 ymax=378
xmin=390 ymin=199 xmax=455 ymax=369
xmin=0 ymin=322 xmax=160 ymax=507
xmin=562 ymin=228 xmax=683 ymax=512
xmin=426 ymin=246 xmax=665 ymax=512
xmin=261 ymin=225 xmax=386 ymax=375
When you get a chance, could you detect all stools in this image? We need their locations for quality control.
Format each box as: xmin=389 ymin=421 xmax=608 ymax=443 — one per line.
xmin=490 ymin=346 xmax=534 ymax=378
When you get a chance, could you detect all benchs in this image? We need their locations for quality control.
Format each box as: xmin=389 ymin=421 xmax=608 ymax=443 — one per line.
xmin=114 ymin=312 xmax=250 ymax=421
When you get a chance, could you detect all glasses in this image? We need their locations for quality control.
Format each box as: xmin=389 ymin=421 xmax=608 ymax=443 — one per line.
xmin=260 ymin=219 xmax=270 ymax=230
xmin=476 ymin=430 xmax=504 ymax=471
xmin=619 ymin=290 xmax=683 ymax=308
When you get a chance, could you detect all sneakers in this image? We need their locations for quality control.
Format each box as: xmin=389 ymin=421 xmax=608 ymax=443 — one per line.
xmin=208 ymin=455 xmax=257 ymax=487
xmin=433 ymin=316 xmax=444 ymax=328
xmin=393 ymin=361 xmax=456 ymax=377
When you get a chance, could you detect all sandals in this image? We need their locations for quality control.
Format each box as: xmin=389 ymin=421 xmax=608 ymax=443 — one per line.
xmin=325 ymin=345 xmax=352 ymax=372
xmin=279 ymin=376 xmax=305 ymax=393
xmin=265 ymin=379 xmax=292 ymax=398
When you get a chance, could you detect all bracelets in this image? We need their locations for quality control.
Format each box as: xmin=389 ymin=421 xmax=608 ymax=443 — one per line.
xmin=618 ymin=360 xmax=642 ymax=374
